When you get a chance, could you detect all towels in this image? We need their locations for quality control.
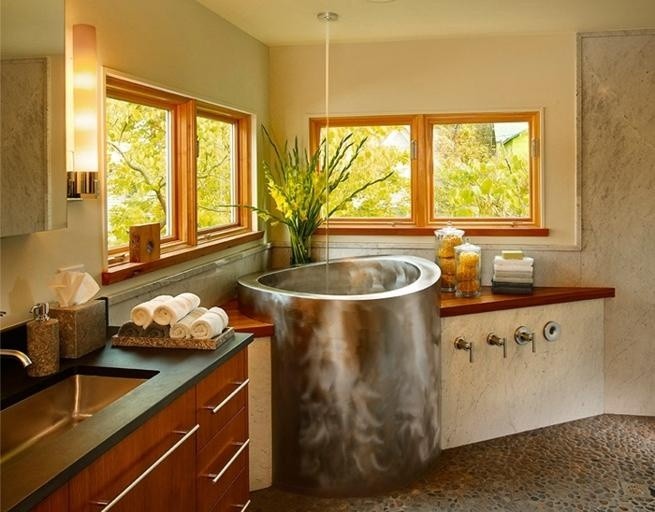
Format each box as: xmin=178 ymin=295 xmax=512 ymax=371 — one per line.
xmin=118 ymin=293 xmax=230 ymax=338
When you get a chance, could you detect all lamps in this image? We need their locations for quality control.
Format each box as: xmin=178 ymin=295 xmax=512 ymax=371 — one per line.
xmin=71 ymin=24 xmax=100 ymax=198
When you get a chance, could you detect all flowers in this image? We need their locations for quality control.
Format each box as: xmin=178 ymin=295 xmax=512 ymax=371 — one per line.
xmin=212 ymin=120 xmax=393 ymax=267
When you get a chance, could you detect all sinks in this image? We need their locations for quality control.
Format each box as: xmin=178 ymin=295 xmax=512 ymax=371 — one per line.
xmin=0 ymin=365 xmax=161 ymax=471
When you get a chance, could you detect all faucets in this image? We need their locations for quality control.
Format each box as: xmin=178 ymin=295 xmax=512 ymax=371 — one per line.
xmin=0 ymin=347 xmax=32 ymax=370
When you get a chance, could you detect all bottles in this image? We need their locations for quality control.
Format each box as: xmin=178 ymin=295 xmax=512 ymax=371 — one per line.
xmin=433 ymin=219 xmax=483 ymax=300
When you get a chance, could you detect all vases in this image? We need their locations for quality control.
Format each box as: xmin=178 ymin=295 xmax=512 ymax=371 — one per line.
xmin=289 ymin=234 xmax=313 ymax=268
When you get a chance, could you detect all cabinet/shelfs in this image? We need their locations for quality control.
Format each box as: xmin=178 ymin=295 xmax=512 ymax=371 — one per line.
xmin=29 ymin=346 xmax=248 ymax=511
